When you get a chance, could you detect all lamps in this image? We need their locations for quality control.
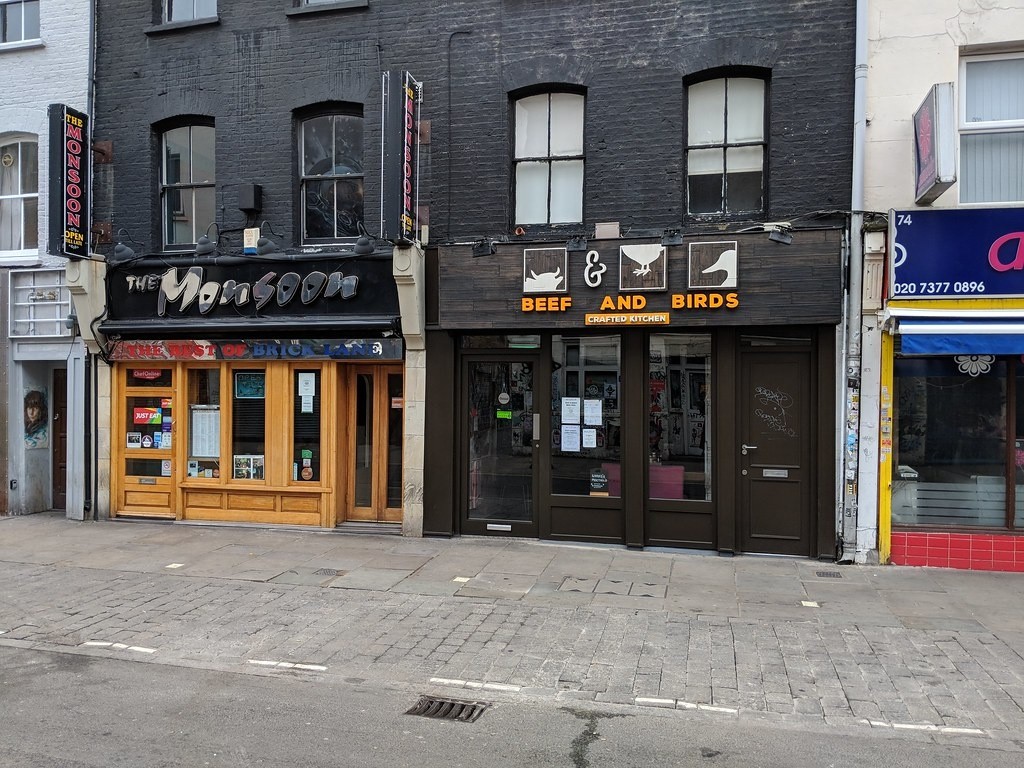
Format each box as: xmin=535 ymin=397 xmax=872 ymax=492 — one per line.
xmin=114 ymin=228 xmax=143 ymax=264
xmin=471 ymin=239 xmax=496 ymax=259
xmin=196 ymin=222 xmax=228 ymax=254
xmin=257 ymin=221 xmax=283 ymax=255
xmin=660 ymin=229 xmax=683 ymax=246
xmin=769 ymin=226 xmax=794 ymax=246
xmin=354 ymin=221 xmax=378 ymax=255
xmin=566 ymin=239 xmax=588 ymax=252
xmin=64 ymin=314 xmax=81 ymax=336
